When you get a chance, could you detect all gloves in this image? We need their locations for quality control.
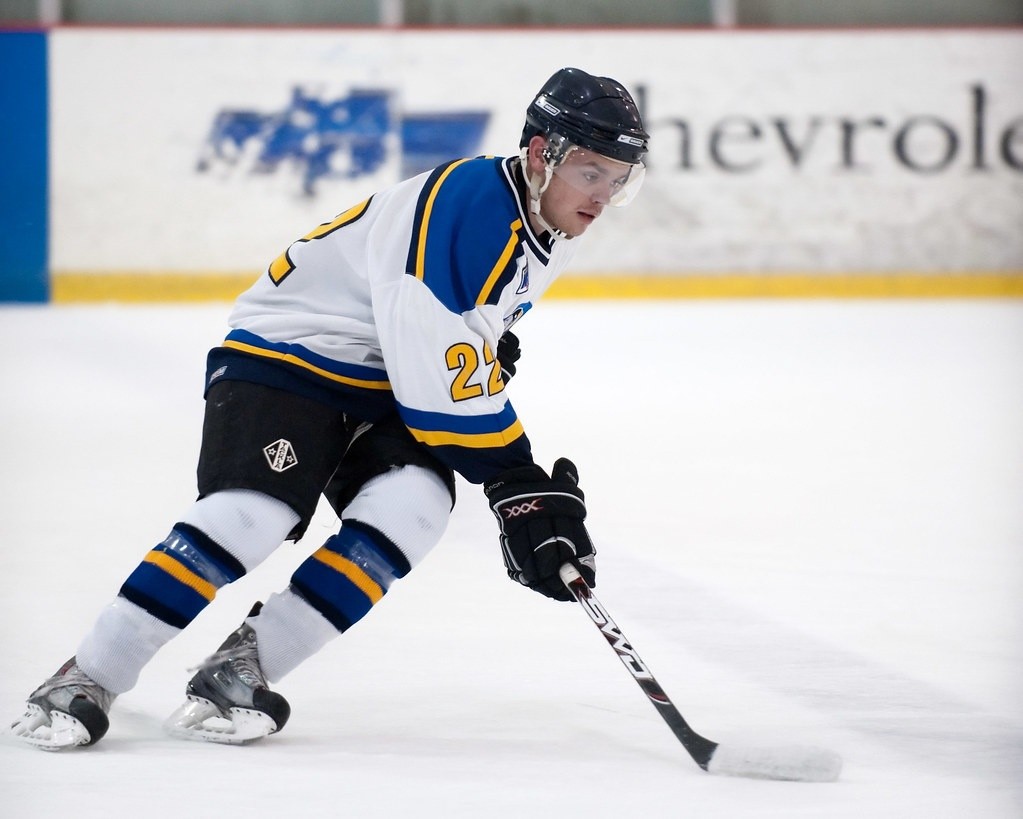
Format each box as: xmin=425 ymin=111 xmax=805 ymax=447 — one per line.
xmin=495 ymin=330 xmax=521 ymax=384
xmin=483 ymin=457 xmax=596 ymax=603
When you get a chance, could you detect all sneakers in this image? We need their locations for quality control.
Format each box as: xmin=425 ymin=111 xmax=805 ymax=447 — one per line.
xmin=9 ymin=656 xmax=117 ymax=750
xmin=165 ymin=601 xmax=291 ymax=743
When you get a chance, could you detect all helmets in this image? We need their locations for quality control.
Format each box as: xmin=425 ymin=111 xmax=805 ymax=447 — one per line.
xmin=519 ymin=66 xmax=650 ymax=169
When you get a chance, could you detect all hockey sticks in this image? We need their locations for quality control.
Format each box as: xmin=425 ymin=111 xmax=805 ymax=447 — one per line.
xmin=558 ymin=561 xmax=852 ymax=784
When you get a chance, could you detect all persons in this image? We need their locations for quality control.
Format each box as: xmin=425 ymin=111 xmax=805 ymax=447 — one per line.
xmin=26 ymin=66 xmax=647 ymax=745
